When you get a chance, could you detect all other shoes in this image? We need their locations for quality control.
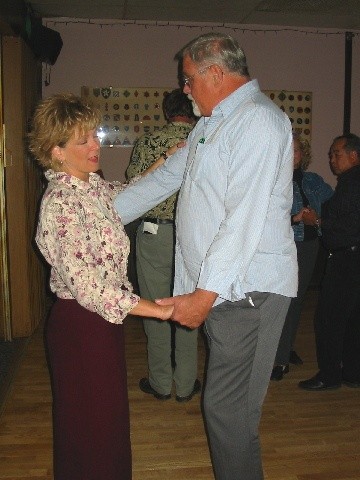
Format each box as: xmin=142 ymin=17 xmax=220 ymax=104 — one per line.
xmin=176 ymin=379 xmax=200 ymax=402
xmin=139 ymin=377 xmax=171 ymax=400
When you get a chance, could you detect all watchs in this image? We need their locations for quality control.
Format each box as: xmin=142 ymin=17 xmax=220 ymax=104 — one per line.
xmin=313 ymin=217 xmax=319 ymax=229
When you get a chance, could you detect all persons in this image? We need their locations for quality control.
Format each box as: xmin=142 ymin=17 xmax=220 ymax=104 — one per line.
xmin=27 ymin=94 xmax=186 ymax=480
xmin=116 ymin=36 xmax=300 ymax=480
xmin=125 ymin=88 xmax=201 ymax=401
xmin=298 ymin=135 xmax=360 ymax=389
xmin=271 ymin=132 xmax=336 ymax=381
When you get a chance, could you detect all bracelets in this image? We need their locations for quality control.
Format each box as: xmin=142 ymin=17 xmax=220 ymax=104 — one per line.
xmin=158 ymin=150 xmax=167 ymax=160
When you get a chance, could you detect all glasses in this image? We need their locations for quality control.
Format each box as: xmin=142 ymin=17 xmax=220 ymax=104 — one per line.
xmin=184 ymin=65 xmax=223 ymax=87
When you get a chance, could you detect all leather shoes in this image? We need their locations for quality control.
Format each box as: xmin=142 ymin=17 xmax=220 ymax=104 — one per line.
xmin=287 ymin=350 xmax=304 ymax=364
xmin=298 ymin=372 xmax=340 ymax=391
xmin=270 ymin=363 xmax=290 ymax=382
xmin=340 ymin=368 xmax=360 ymax=388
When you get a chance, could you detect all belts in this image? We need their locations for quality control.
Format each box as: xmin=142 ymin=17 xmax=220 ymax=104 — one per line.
xmin=143 ymin=217 xmax=175 ymax=225
xmin=334 ymin=246 xmax=360 ymax=256
